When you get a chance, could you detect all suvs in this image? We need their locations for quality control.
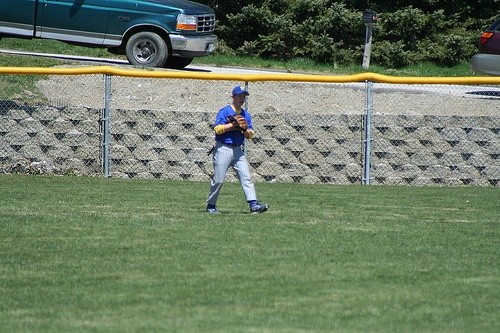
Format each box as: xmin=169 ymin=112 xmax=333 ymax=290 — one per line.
xmin=0 ymin=0 xmax=219 ymax=68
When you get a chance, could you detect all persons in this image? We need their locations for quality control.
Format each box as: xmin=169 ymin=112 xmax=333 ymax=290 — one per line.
xmin=205 ymin=86 xmax=270 ymax=215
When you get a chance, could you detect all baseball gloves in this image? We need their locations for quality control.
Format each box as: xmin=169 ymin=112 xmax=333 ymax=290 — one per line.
xmin=227 ymin=115 xmax=247 ymax=132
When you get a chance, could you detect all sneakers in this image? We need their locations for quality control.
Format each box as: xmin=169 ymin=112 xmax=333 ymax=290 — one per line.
xmin=249 ymin=203 xmax=269 ymax=214
xmin=206 ymin=205 xmax=221 ymax=214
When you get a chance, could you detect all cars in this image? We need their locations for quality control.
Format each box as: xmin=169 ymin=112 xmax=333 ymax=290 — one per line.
xmin=471 ymin=17 xmax=500 ymax=76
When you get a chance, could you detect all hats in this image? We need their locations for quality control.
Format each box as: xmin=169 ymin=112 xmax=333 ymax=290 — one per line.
xmin=232 ymin=86 xmax=249 ymax=96
